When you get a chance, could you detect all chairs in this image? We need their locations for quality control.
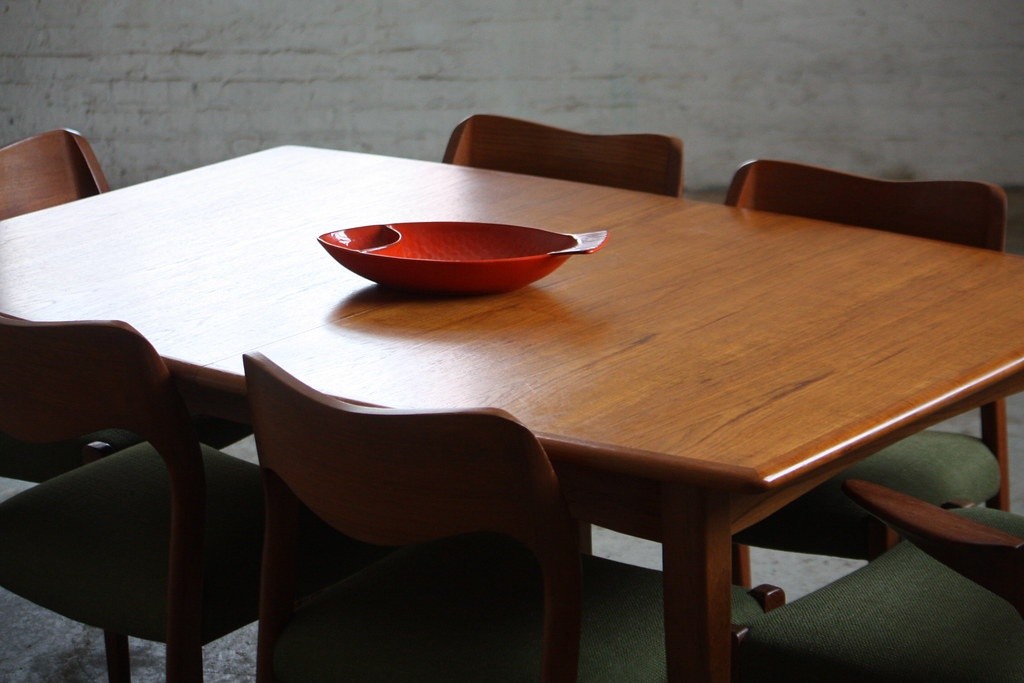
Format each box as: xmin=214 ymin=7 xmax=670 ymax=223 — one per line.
xmin=0 ymin=126 xmax=252 ymax=482
xmin=240 ymin=352 xmax=785 ymax=683
xmin=0 ymin=316 xmax=260 ymax=683
xmin=724 ymin=158 xmax=1007 ymax=587
xmin=442 ymin=114 xmax=684 ymax=199
xmin=729 ymin=507 xmax=1024 ymax=682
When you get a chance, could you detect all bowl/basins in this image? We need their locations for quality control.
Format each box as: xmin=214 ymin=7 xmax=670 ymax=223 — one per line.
xmin=315 ymin=221 xmax=612 ymax=296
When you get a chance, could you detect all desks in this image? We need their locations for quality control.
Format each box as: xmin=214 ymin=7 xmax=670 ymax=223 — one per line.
xmin=0 ymin=145 xmax=1023 ymax=683
